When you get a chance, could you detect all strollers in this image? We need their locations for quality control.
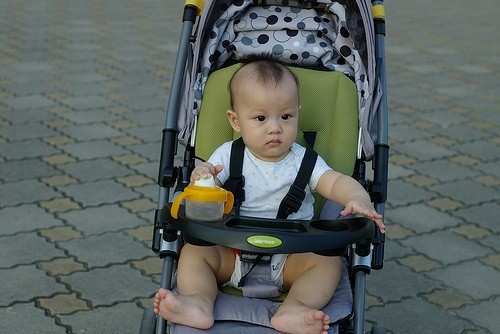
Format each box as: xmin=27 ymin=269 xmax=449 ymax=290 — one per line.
xmin=141 ymin=0 xmax=391 ymax=333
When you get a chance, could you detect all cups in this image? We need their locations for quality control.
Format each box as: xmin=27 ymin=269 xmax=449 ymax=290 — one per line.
xmin=170 ymin=173 xmax=234 ymax=222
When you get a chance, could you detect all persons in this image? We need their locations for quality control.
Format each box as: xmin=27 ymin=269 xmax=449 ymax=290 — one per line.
xmin=152 ymin=53 xmax=385 ymax=334
